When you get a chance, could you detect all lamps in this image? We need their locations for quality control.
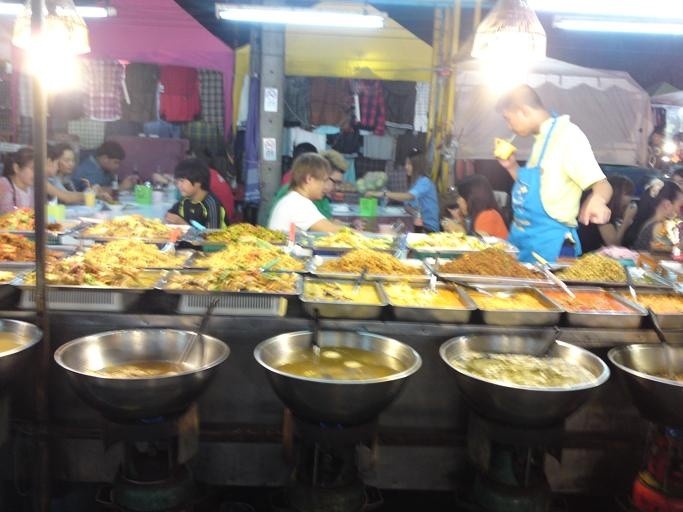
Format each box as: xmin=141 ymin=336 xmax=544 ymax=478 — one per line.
xmin=213 ymin=2 xmax=385 ymax=30
xmin=553 ymin=13 xmax=683 ymax=36
xmin=471 ymin=1 xmax=546 ymax=84
xmin=0 ymin=2 xmax=118 ymax=18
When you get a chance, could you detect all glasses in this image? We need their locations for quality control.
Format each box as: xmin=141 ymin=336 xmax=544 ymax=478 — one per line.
xmin=408 ymin=147 xmax=418 ymax=152
xmin=329 ymin=177 xmax=342 ymax=187
xmin=447 ymin=185 xmax=460 ymax=196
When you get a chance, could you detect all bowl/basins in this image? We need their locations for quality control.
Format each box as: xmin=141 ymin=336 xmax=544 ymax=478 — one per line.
xmin=54 ymin=329 xmax=232 ymax=419
xmin=253 ymin=330 xmax=423 ymax=425
xmin=607 ymin=343 xmax=683 ymax=428
xmin=438 ymin=335 xmax=611 ymax=426
xmin=0 ymin=316 xmax=44 ymax=387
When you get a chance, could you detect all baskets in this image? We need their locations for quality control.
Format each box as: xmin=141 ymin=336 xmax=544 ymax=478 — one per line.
xmin=175 ymin=292 xmax=288 ymax=318
xmin=21 ymin=286 xmax=140 ymax=312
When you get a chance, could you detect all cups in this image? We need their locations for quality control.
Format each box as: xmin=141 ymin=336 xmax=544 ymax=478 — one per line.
xmin=494 ymin=138 xmax=516 ymax=161
xmin=152 ymin=191 xmax=162 ymax=203
xmin=83 ymin=188 xmax=96 ymax=207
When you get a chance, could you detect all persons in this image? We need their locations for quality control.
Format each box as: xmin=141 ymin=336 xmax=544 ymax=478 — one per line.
xmin=0 ymin=139 xmax=139 ymax=216
xmin=262 ymin=104 xmax=362 ymax=236
xmin=441 ymin=176 xmax=508 ymax=238
xmin=492 ymin=85 xmax=614 ymax=264
xmin=577 ymin=133 xmax=683 ymax=257
xmin=152 ymin=147 xmax=235 ymax=230
xmin=362 ymin=146 xmax=441 ymax=232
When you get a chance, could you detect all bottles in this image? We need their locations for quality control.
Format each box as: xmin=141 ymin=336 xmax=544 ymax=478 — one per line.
xmin=112 ymin=181 xmax=119 ymax=201
xmin=167 ymin=179 xmax=176 ymax=202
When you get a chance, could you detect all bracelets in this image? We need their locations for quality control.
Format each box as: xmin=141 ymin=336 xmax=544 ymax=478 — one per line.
xmin=383 ymin=191 xmax=387 ymax=198
